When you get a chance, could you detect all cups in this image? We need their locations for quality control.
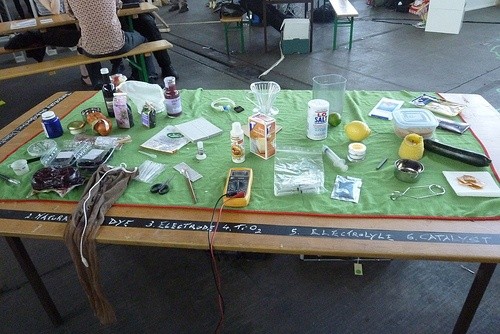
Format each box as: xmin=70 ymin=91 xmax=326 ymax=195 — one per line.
xmin=312 ymin=74 xmax=348 ymax=115
xmin=250 ymin=81 xmax=281 ymax=116
xmin=306 ymin=99 xmax=329 ymax=140
xmin=11 ymin=159 xmax=29 ymax=175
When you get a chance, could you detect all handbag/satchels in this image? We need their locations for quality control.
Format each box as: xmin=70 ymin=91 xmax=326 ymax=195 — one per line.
xmin=314 ymin=5 xmax=333 ymax=22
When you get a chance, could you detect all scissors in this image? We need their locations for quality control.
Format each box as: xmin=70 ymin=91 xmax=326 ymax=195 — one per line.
xmin=151 ymin=173 xmax=176 ymax=194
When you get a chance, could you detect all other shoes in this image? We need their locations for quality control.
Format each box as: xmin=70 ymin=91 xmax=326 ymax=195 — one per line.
xmin=148 ymin=73 xmax=157 ymax=78
xmin=163 ymin=65 xmax=179 ymax=79
xmin=169 ymin=4 xmax=179 ymax=12
xmin=179 ymin=6 xmax=189 ymax=13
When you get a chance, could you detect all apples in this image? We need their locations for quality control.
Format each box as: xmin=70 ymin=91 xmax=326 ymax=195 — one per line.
xmin=87 ymin=112 xmax=112 ymax=136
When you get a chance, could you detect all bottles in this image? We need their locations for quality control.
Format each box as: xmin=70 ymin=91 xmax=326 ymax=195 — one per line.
xmin=40 ymin=110 xmax=63 ymax=139
xmin=161 ymin=76 xmax=182 ymax=119
xmin=230 ymin=121 xmax=246 ymax=164
xmin=100 ymin=68 xmax=116 ymax=118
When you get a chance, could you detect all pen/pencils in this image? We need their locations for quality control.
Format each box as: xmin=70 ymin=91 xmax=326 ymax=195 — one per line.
xmin=8 ymin=157 xmax=41 ymax=168
xmin=185 ymin=170 xmax=197 ymax=202
xmin=0 ymin=174 xmax=20 ymax=185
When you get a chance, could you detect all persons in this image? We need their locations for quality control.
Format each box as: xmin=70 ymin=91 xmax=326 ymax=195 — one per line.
xmin=168 ymin=0 xmax=189 ymax=13
xmin=33 ymin=0 xmax=178 ymax=88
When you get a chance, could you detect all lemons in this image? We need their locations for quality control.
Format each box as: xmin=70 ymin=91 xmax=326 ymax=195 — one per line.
xmin=344 ymin=121 xmax=371 ymax=141
xmin=328 ymin=112 xmax=342 ymax=127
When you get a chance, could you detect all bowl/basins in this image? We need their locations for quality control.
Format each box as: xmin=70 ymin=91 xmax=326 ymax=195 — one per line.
xmin=395 ymin=159 xmax=424 ymax=183
xmin=392 ymin=108 xmax=440 ymax=140
xmin=81 ymin=107 xmax=101 ymax=122
xmin=40 ymin=139 xmax=118 ymax=169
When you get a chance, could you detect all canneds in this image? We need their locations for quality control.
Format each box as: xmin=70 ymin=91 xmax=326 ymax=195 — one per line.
xmin=307 ymin=99 xmax=329 ymax=140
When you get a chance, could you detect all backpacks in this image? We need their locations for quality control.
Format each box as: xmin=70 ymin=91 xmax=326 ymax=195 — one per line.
xmin=221 ymin=3 xmax=246 ymax=16
xmin=373 ymin=0 xmax=410 ymax=12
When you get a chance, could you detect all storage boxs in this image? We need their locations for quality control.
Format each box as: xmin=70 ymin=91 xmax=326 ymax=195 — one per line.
xmin=281 ymin=39 xmax=310 ymax=55
xmin=392 ymin=108 xmax=439 ymax=140
xmin=248 ymin=112 xmax=278 ymax=160
xmin=280 ymin=17 xmax=310 ymax=40
xmin=141 ymin=107 xmax=157 ymax=128
xmin=111 ymin=93 xmax=135 ymax=129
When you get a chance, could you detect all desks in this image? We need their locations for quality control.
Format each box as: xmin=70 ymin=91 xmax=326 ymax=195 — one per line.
xmin=0 ymin=2 xmax=161 ymax=39
xmin=0 ymin=86 xmax=500 ymax=334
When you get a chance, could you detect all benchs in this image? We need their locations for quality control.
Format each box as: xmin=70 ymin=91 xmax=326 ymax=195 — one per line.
xmin=220 ymin=13 xmax=244 ymax=56
xmin=0 ymin=40 xmax=173 ymax=93
xmin=329 ymin=0 xmax=359 ymax=51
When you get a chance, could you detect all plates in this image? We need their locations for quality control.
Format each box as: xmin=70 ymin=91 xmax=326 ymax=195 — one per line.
xmin=27 ymin=139 xmax=57 ymax=156
